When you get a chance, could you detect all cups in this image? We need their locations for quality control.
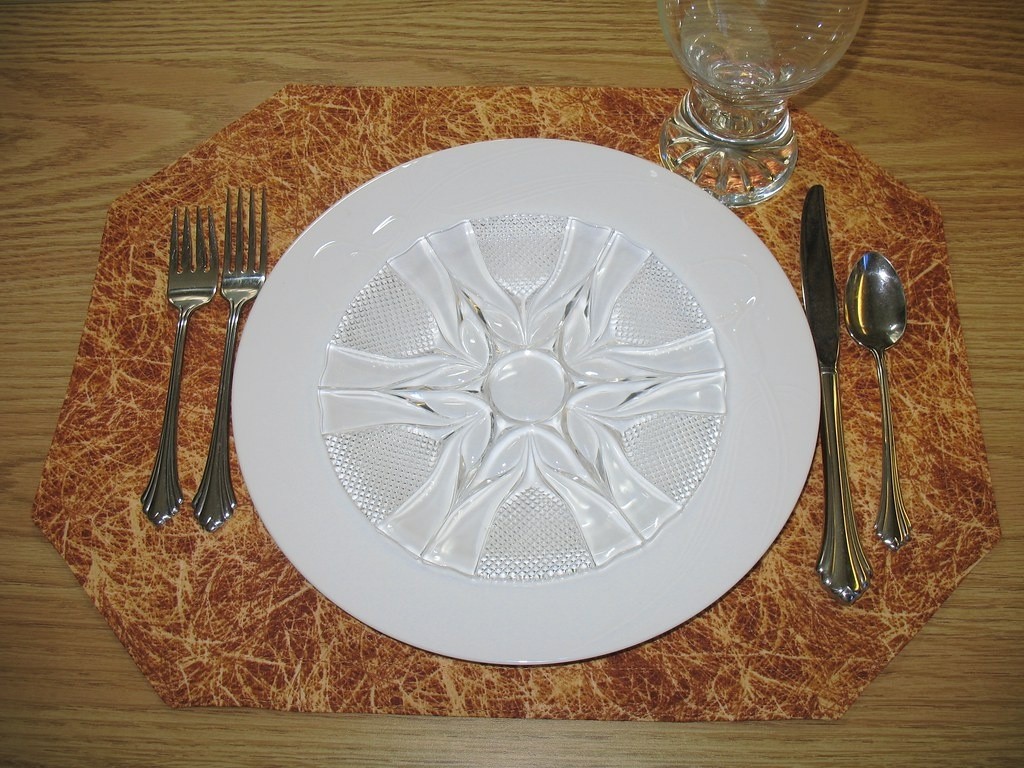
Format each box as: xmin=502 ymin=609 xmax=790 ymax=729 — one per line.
xmin=657 ymin=0 xmax=866 ymax=209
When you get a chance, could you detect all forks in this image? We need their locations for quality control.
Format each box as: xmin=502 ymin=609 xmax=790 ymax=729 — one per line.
xmin=190 ymin=187 xmax=269 ymax=533
xmin=140 ymin=204 xmax=218 ymax=527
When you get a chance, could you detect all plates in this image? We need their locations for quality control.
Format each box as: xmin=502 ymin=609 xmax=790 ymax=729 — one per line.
xmin=232 ymin=134 xmax=823 ymax=669
xmin=317 ymin=210 xmax=730 ymax=583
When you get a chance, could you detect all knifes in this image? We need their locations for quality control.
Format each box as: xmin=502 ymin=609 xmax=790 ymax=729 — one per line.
xmin=801 ymin=181 xmax=872 ymax=601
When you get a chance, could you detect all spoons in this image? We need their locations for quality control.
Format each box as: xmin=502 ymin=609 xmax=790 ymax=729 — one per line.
xmin=844 ymin=250 xmax=912 ymax=548
